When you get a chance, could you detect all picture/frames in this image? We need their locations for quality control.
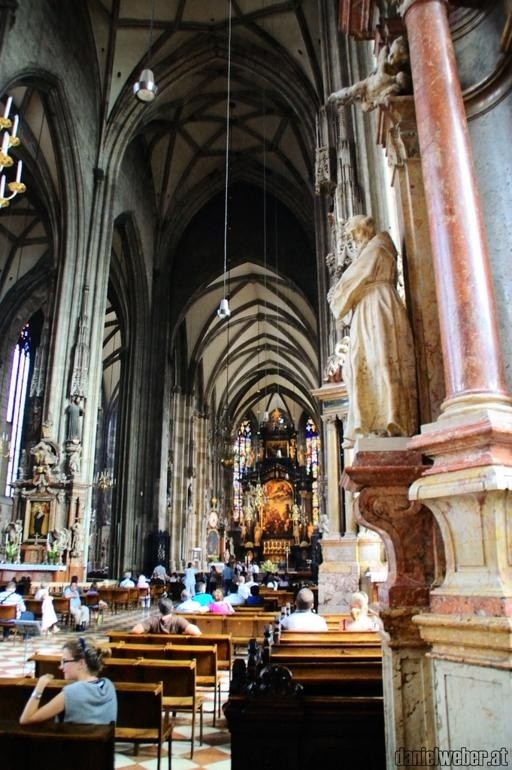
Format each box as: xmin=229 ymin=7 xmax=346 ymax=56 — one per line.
xmin=20 ymin=497 xmax=57 ymax=543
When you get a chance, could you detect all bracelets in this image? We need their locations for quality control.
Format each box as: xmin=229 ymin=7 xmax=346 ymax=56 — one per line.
xmin=32 ymin=689 xmax=42 ymax=700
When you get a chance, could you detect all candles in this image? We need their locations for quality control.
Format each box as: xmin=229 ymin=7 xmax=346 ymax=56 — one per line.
xmin=0 ymin=93 xmax=24 ymax=201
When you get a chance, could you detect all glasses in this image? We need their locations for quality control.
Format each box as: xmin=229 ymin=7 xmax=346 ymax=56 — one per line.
xmin=60 ymin=657 xmax=77 ymax=666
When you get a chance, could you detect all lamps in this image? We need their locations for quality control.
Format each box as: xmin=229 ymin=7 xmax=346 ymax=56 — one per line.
xmin=132 ymin=1 xmax=158 ymax=105
xmin=203 ymin=1 xmax=270 ymax=423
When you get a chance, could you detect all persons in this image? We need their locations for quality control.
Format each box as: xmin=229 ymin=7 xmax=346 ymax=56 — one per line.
xmin=131 ymin=599 xmax=206 ymax=635
xmin=62 ymin=581 xmax=90 ymax=631
xmin=89 ymin=579 xmax=112 ymax=623
xmin=0 ymin=580 xmax=32 ymax=642
xmin=339 ymin=592 xmax=386 ymax=631
xmin=34 ymin=582 xmax=61 ymax=636
xmin=316 ymin=34 xmax=409 ymax=114
xmin=115 ymin=560 xmax=291 ymax=614
xmin=32 ymin=504 xmax=46 ymax=538
xmin=18 ymin=637 xmax=118 ymax=730
xmin=69 ymin=518 xmax=85 ymax=553
xmin=275 ymin=586 xmax=328 ymax=633
xmin=325 ymin=212 xmax=422 ymax=440
